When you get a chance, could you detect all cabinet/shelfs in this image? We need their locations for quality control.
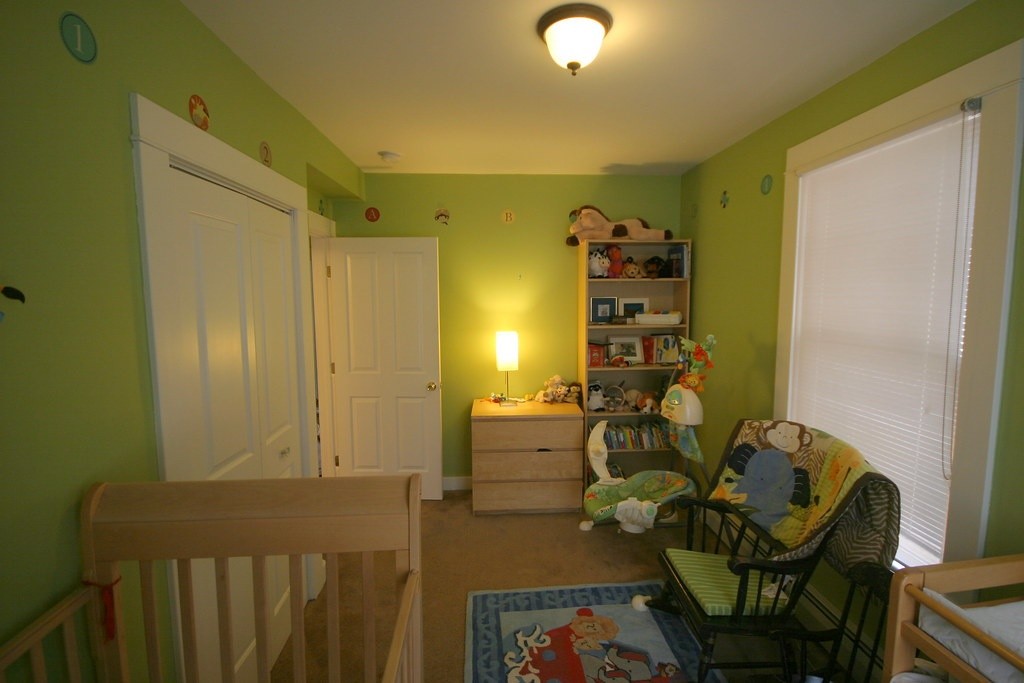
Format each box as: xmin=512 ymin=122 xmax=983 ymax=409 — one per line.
xmin=577 ymin=239 xmax=692 ymax=492
xmin=471 ymin=398 xmax=584 ymax=515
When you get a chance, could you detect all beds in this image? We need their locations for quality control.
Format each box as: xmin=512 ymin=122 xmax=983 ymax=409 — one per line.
xmin=883 ymin=554 xmax=1024 ymax=683
xmin=0 ymin=473 xmax=425 ymax=683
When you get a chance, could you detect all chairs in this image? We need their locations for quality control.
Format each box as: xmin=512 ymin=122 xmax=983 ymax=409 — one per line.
xmin=631 ymin=427 xmax=863 ymax=683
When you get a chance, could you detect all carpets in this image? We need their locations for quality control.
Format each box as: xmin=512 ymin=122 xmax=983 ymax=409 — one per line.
xmin=465 ymin=579 xmax=728 ymax=683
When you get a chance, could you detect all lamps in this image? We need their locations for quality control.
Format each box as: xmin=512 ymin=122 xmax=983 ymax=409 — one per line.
xmin=537 ymin=4 xmax=613 ymax=76
xmin=496 ymin=330 xmax=519 ymax=407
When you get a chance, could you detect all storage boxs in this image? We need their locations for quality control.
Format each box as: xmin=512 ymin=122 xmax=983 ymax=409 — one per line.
xmin=636 ymin=312 xmax=682 ymax=324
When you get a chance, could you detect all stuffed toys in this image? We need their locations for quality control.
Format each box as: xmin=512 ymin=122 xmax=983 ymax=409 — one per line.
xmin=535 ymin=375 xmax=581 ymax=404
xmin=608 ymin=246 xmax=681 ymax=277
xmin=566 ymin=206 xmax=673 ymax=247
xmin=606 ymin=381 xmax=661 ymax=414
xmin=588 ymin=380 xmax=606 ymax=411
xmin=589 ymin=248 xmax=610 ymax=278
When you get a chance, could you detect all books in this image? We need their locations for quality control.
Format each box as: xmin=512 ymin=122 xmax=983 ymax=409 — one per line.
xmin=604 ymin=422 xmax=671 ymax=449
xmin=588 ymin=465 xmax=624 ymax=486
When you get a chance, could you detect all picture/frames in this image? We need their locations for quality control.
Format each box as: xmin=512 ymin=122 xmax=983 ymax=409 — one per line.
xmin=590 ymin=297 xmax=618 ymax=323
xmin=618 ymin=298 xmax=648 ymax=323
xmin=607 ymin=336 xmax=644 ymax=364
